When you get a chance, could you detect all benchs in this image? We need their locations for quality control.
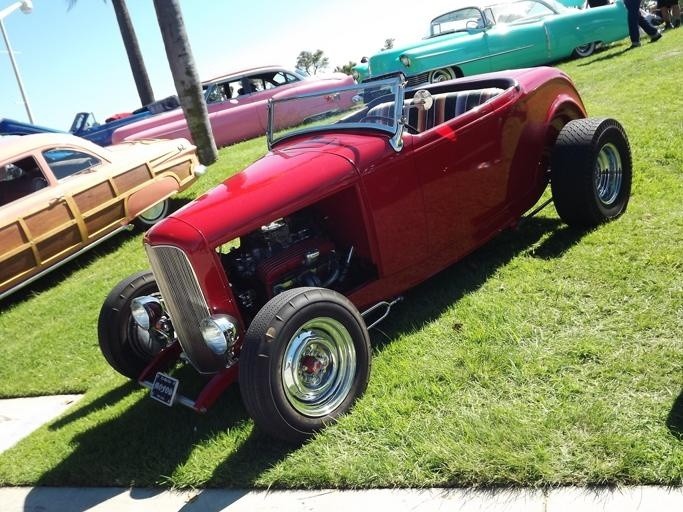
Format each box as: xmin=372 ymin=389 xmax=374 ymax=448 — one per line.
xmin=364 ymin=87 xmax=504 ymax=132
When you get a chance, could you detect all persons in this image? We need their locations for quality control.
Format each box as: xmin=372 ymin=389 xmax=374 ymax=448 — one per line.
xmin=655 ymin=0 xmax=682 ymax=32
xmin=622 ymin=0 xmax=662 ymax=51
xmin=584 ymin=0 xmax=611 ymax=10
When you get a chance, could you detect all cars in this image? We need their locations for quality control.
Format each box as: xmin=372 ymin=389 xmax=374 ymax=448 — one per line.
xmin=97 ymin=65 xmax=631 ymax=442
xmin=0 ymin=132 xmax=206 ymax=302
xmin=351 ymin=0 xmax=628 ymax=106
xmin=71 ymin=63 xmax=359 ymax=146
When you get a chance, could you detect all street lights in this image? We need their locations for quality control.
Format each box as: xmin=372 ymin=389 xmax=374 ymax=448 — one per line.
xmin=0 ymin=0 xmax=34 ymax=125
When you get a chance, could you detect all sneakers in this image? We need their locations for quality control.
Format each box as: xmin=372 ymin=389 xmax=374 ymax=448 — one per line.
xmin=625 ymin=32 xmax=662 ymax=51
